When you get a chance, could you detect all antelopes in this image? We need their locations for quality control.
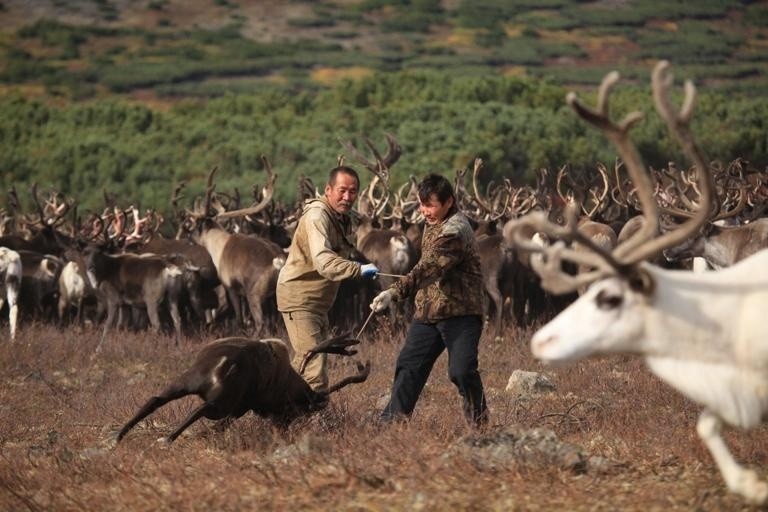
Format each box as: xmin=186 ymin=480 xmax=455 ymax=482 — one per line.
xmin=2 ymin=134 xmax=767 ymax=338
xmin=104 ymin=325 xmax=373 ymax=458
xmin=503 ymin=62 xmax=766 ymax=508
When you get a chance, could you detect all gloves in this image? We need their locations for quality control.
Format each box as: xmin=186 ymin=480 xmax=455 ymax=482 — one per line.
xmin=361 ymin=263 xmax=379 ymax=281
xmin=369 ymin=288 xmax=396 ymax=313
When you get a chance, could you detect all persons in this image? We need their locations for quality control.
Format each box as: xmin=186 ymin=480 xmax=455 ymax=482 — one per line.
xmin=368 ymin=172 xmax=492 ymax=431
xmin=275 ymin=166 xmax=382 ymax=401
